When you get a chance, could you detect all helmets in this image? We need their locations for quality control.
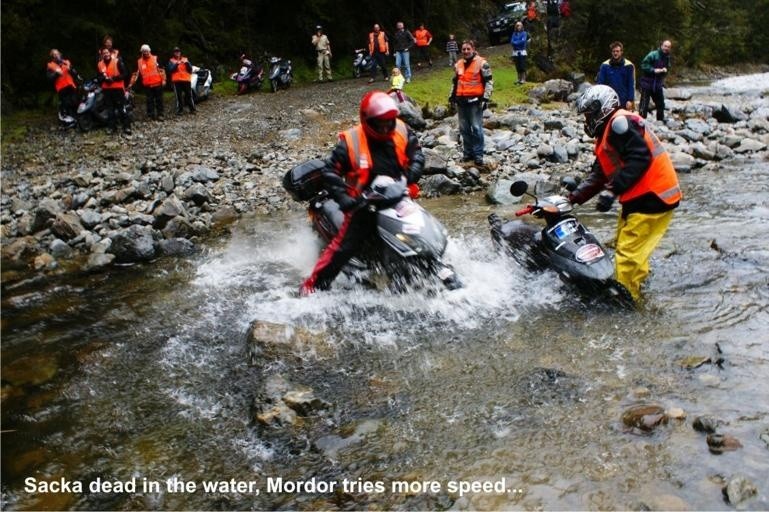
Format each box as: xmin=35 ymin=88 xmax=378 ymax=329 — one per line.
xmin=360 ymin=90 xmax=400 ymax=142
xmin=575 ymin=82 xmax=621 ymax=139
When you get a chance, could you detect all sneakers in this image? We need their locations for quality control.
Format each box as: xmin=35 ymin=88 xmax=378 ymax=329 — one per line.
xmin=104 ymin=125 xmax=132 ymax=135
xmin=146 ymin=112 xmax=165 ymax=123
xmin=459 ymin=155 xmax=483 ymax=167
xmin=177 ymin=107 xmax=197 ymax=114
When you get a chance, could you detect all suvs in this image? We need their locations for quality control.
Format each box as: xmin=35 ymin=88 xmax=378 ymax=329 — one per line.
xmin=486 ymin=0 xmax=548 ymax=46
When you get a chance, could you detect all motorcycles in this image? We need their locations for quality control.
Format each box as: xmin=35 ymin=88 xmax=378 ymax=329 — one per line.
xmin=284 ymin=153 xmax=465 ymax=304
xmin=74 ymin=74 xmax=134 ymax=134
xmin=189 ymin=64 xmax=215 ymax=105
xmin=487 ymin=173 xmax=638 ymax=318
xmin=263 ymin=51 xmax=295 ymax=94
xmin=350 ymin=46 xmax=383 ymax=78
xmin=232 ymin=53 xmax=264 ymax=96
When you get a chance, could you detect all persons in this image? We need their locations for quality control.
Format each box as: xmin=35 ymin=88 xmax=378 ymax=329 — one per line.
xmin=298 ymin=89 xmax=426 ymax=298
xmin=311 ymin=25 xmax=334 ymax=81
xmin=391 ymin=20 xmax=417 ymax=84
xmin=386 ymin=68 xmax=405 ymax=102
xmin=367 ymin=23 xmax=390 ymax=84
xmin=46 ymin=47 xmax=83 ymax=130
xmin=445 ymin=32 xmax=459 ymax=67
xmin=448 ymin=39 xmax=494 ymax=167
xmin=567 ymin=84 xmax=683 ymax=301
xmin=510 ymin=21 xmax=527 ymax=86
xmin=413 ymin=24 xmax=433 ymax=70
xmin=636 ymin=39 xmax=673 ymax=124
xmin=595 ymin=40 xmax=636 ymax=112
xmin=94 ymin=35 xmax=197 ymax=135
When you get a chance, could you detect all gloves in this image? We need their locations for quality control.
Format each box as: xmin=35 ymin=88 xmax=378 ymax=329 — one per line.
xmin=337 ymin=190 xmax=356 ymax=214
xmin=404 ymin=171 xmax=420 ymax=186
xmin=596 ymin=191 xmax=613 ymax=212
xmin=480 ymin=98 xmax=489 ymax=112
